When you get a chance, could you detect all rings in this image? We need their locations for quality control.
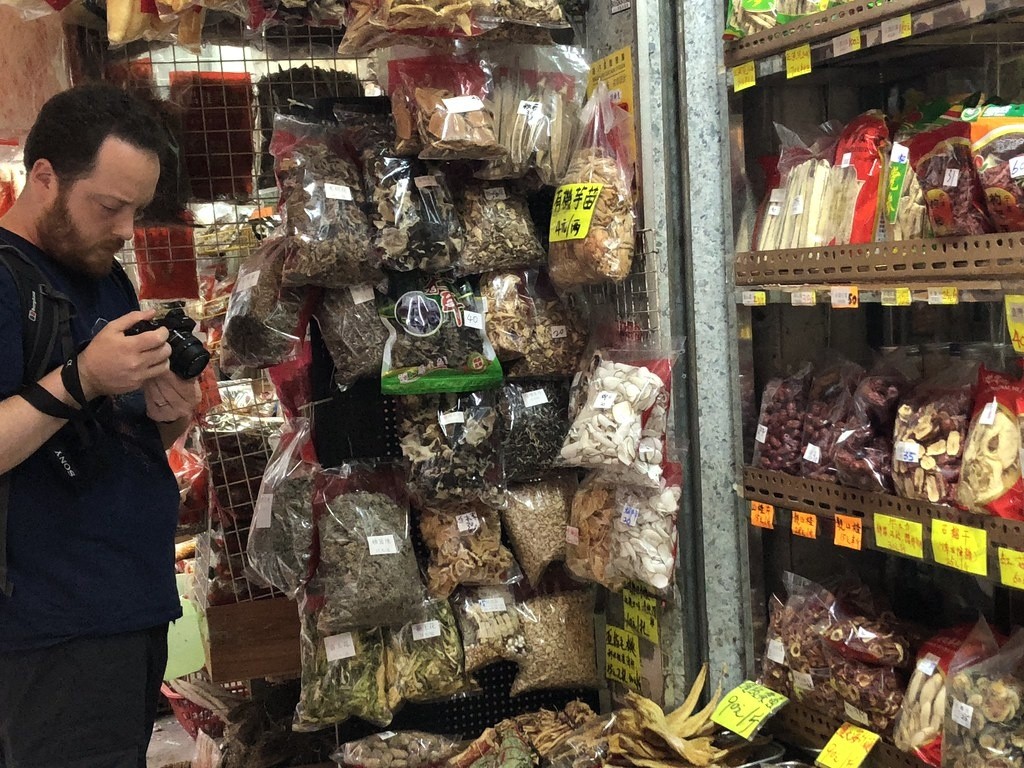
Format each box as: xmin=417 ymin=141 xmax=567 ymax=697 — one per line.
xmin=159 ymin=402 xmax=167 ymax=406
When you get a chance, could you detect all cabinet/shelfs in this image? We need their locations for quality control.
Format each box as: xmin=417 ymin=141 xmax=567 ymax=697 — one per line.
xmin=722 ymin=0 xmax=1024 ymax=768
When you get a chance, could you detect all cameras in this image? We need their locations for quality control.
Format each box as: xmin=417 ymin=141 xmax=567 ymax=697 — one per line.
xmin=124 ymin=308 xmax=210 ymax=379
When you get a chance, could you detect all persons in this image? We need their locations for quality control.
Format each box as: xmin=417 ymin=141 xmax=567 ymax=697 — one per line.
xmin=0 ymin=81 xmax=203 ymax=768
